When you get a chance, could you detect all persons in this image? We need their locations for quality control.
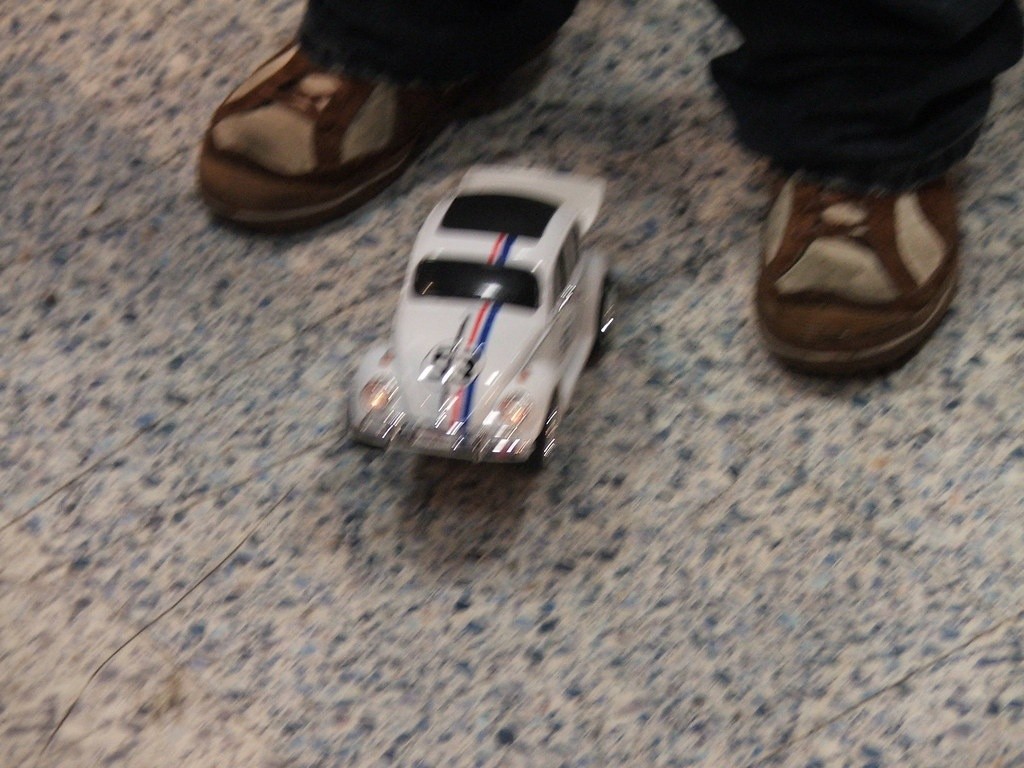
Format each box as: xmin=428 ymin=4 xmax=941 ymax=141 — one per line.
xmin=199 ymin=0 xmax=1023 ymax=379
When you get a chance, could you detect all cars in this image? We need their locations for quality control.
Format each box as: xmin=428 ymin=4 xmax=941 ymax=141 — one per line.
xmin=347 ymin=161 xmax=620 ymax=471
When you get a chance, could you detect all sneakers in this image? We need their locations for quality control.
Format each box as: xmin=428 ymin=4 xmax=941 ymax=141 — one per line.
xmin=753 ymin=166 xmax=958 ymax=375
xmin=194 ymin=33 xmax=443 ymax=230
